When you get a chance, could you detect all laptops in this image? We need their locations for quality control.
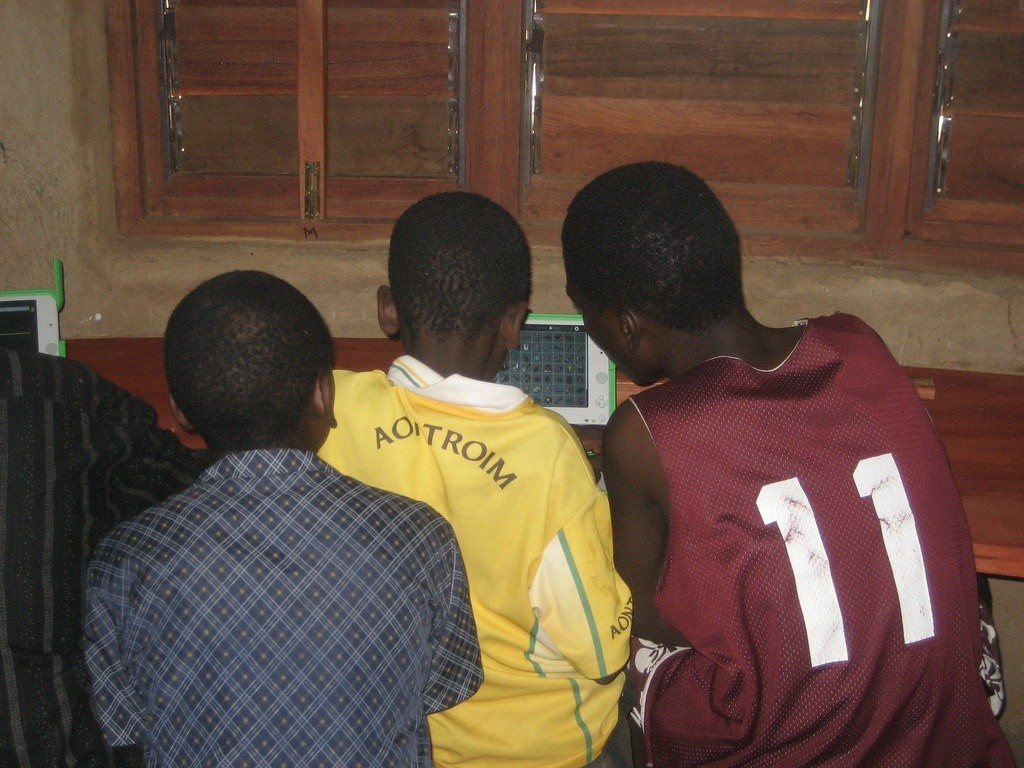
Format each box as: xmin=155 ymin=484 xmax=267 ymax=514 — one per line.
xmin=490 ymin=313 xmax=618 ymax=494
xmin=0 ymin=256 xmax=67 ymax=359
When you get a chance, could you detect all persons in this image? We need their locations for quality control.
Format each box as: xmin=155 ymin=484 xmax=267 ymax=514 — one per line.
xmin=311 ymin=192 xmax=633 ymax=768
xmin=0 ymin=343 xmax=209 ymax=768
xmin=561 ymin=161 xmax=1017 ymax=768
xmin=80 ymin=269 xmax=484 ymax=768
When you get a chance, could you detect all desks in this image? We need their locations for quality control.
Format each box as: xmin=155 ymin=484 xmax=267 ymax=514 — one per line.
xmin=64 ymin=335 xmax=1024 ymax=579
